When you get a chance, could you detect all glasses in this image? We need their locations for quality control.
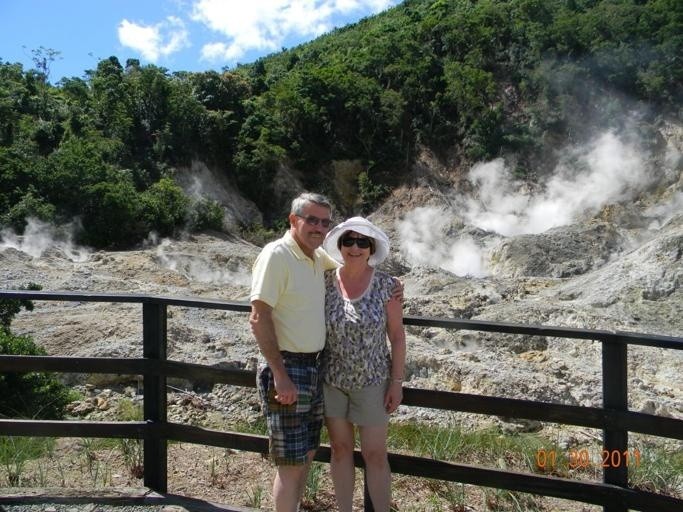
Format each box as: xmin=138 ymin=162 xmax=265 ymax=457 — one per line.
xmin=294 ymin=213 xmax=330 ymax=229
xmin=341 ymin=236 xmax=371 ymax=249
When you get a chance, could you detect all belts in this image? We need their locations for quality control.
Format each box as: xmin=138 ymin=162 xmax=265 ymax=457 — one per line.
xmin=279 ymin=351 xmax=323 ymax=364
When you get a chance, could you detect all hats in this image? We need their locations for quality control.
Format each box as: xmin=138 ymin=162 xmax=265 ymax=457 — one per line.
xmin=322 ymin=216 xmax=390 ymax=266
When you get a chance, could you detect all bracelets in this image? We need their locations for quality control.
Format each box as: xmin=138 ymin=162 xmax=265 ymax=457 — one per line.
xmin=388 ymin=374 xmax=403 ymax=384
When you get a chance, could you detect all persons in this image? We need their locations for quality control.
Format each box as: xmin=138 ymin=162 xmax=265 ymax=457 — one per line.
xmin=246 ymin=192 xmax=404 ymax=512
xmin=317 ymin=213 xmax=404 ymax=512
